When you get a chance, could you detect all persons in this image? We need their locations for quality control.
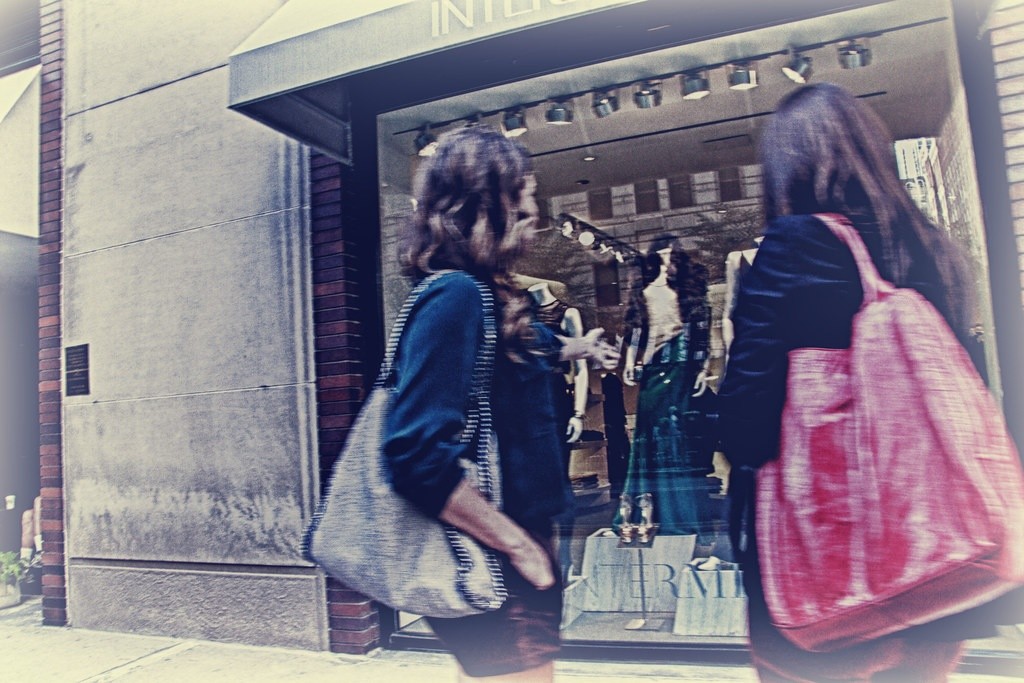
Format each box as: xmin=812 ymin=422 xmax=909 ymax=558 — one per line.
xmin=690 ymin=79 xmax=998 ymax=679
xmin=524 ymin=279 xmax=589 ymax=564
xmin=370 ymin=122 xmax=573 ymax=682
xmin=607 ymin=232 xmax=716 ymax=535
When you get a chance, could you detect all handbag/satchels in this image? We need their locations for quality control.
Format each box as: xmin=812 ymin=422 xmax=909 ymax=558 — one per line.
xmin=303 ymin=270 xmax=509 ymax=617
xmin=751 ymin=212 xmax=1022 ymax=652
